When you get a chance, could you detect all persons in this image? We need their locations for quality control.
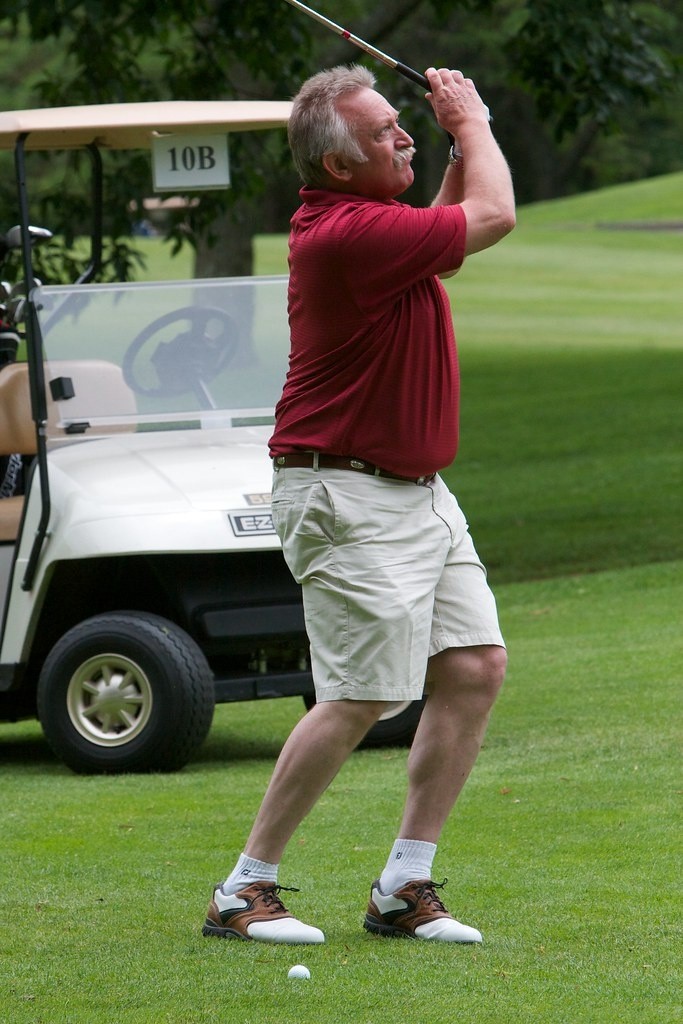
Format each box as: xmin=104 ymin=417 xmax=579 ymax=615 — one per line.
xmin=202 ymin=62 xmax=516 ymax=945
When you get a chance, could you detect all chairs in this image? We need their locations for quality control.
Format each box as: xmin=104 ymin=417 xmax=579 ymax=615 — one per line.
xmin=1 ymin=362 xmax=135 ymax=544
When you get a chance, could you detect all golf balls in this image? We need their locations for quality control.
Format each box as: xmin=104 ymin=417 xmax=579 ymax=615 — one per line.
xmin=287 ymin=965 xmax=311 ymax=979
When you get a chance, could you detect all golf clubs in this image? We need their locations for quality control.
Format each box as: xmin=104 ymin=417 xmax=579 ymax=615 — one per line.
xmin=287 ymin=0 xmax=493 ymax=123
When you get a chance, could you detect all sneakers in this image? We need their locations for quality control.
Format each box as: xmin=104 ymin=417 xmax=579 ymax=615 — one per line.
xmin=363 ymin=878 xmax=482 ymax=943
xmin=201 ymin=880 xmax=324 ymax=945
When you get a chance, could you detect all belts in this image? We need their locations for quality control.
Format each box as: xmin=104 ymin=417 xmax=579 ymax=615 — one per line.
xmin=273 ymin=453 xmax=436 ymax=485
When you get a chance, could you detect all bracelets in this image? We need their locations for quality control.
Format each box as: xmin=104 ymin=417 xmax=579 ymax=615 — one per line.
xmin=447 ymin=145 xmax=464 ymax=170
